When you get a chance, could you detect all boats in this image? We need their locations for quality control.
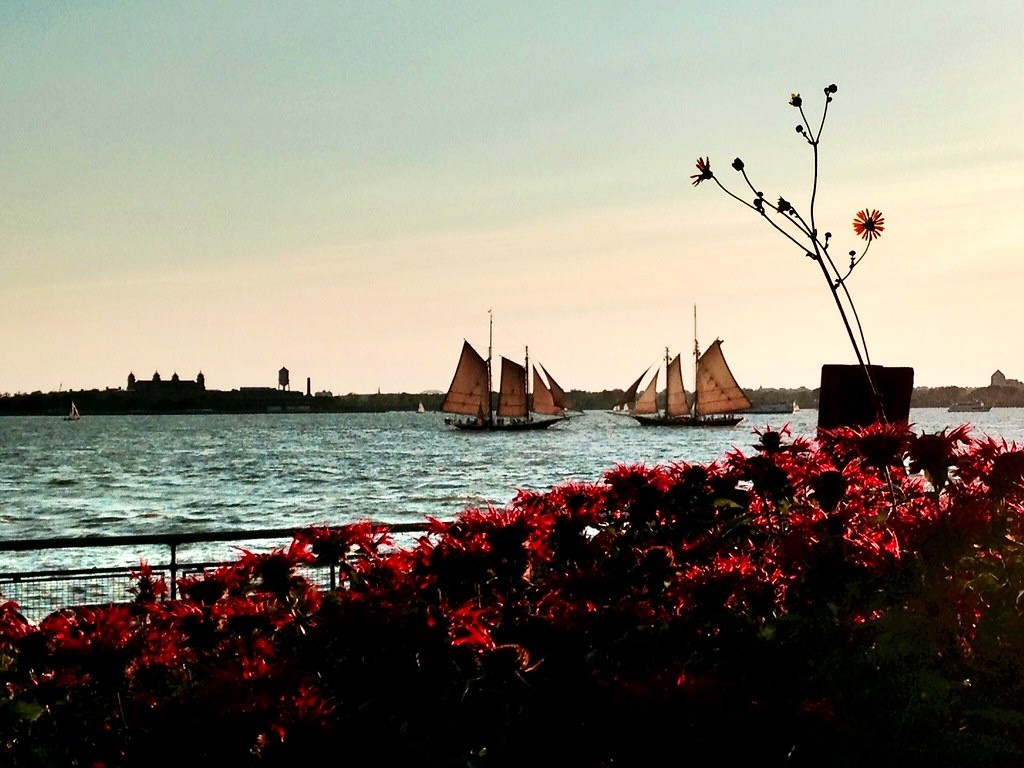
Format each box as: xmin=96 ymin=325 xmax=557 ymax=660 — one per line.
xmin=947 ymin=396 xmax=993 ymax=413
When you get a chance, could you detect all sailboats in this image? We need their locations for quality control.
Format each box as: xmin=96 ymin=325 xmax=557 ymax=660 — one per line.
xmin=63 ymin=400 xmax=81 ymax=420
xmin=416 ymin=400 xmax=426 ymax=414
xmin=604 ymin=301 xmax=753 ymax=427
xmin=440 ymin=307 xmax=592 ymax=432
